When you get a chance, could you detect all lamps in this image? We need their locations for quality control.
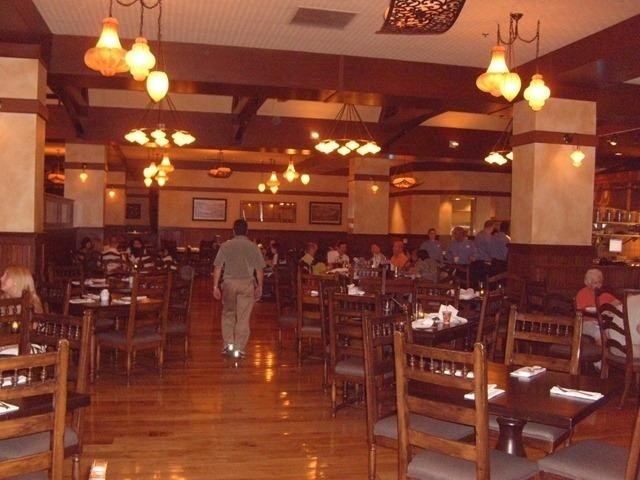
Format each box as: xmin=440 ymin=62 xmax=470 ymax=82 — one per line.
xmin=257 ymin=159 xmax=281 ymax=194
xmin=309 ymin=103 xmax=382 ymax=157
xmin=375 ymin=0 xmax=467 ymax=36
xmin=281 ymin=154 xmax=311 ymax=185
xmin=390 ymin=155 xmax=425 ymax=189
xmin=207 ymin=148 xmax=232 ymax=178
xmin=123 ymin=62 xmax=196 ymax=149
xmin=143 ymin=148 xmax=175 ymax=189
xmin=84 ymin=0 xmax=169 ymax=102
xmin=484 ymin=116 xmax=514 ymax=166
xmin=476 ymin=12 xmax=551 ymax=112
xmin=564 ymin=133 xmax=586 ymax=163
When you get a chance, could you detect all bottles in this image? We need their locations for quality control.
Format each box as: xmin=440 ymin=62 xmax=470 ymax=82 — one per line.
xmin=479 ymin=281 xmax=486 ymax=301
xmin=415 ymin=303 xmax=424 ymax=319
xmin=100 ymin=289 xmax=110 ymax=302
xmin=594 ymin=207 xmax=631 ymax=222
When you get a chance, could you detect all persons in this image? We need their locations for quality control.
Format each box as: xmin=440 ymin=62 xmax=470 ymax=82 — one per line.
xmin=609 ymin=292 xmax=640 ymax=391
xmin=0 ymin=267 xmax=47 ymax=355
xmin=78 ymin=234 xmax=177 ymax=270
xmin=253 ymin=220 xmax=511 ymax=289
xmin=214 ymin=219 xmax=265 ymax=359
xmin=576 ymin=270 xmax=621 ymax=372
xmin=212 ymin=234 xmax=225 ymax=251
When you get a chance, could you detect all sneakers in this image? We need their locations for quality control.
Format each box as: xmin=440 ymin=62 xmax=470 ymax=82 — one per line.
xmin=221 ymin=343 xmax=247 ymax=360
xmin=593 ymin=360 xmax=602 ymax=377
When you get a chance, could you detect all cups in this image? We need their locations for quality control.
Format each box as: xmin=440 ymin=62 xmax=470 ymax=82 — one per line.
xmin=128 ymin=275 xmax=134 ymax=287
xmin=423 ymin=313 xmax=432 ymax=325
xmin=442 ymin=310 xmax=452 ymax=325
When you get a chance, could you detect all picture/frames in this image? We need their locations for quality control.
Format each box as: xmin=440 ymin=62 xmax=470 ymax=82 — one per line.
xmin=309 ymin=200 xmax=342 ymax=226
xmin=239 ymin=200 xmax=297 ymax=224
xmin=191 ymin=197 xmax=228 ymax=222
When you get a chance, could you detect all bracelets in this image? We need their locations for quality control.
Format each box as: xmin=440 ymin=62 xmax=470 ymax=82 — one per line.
xmin=214 ymin=286 xmax=219 ymax=288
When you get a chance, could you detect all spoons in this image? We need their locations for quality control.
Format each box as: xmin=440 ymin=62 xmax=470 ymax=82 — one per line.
xmin=527 ymin=366 xmax=544 ymax=373
xmin=556 ymin=384 xmax=593 ymax=397
